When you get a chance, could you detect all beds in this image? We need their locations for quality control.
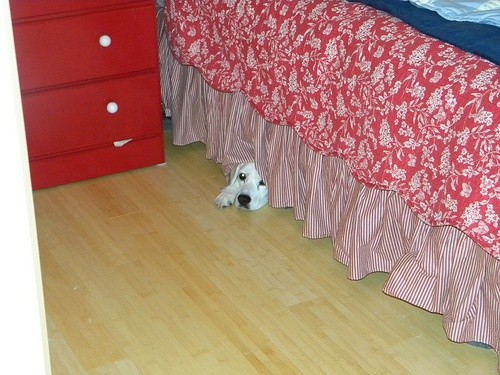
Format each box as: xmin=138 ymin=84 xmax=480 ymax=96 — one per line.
xmin=157 ymin=0 xmax=500 ymax=356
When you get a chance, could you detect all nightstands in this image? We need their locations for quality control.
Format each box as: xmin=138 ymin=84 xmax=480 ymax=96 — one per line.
xmin=9 ymin=0 xmax=165 ymax=192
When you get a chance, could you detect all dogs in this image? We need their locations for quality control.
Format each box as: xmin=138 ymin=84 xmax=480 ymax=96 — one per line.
xmin=215 ymin=162 xmax=269 ymax=210
